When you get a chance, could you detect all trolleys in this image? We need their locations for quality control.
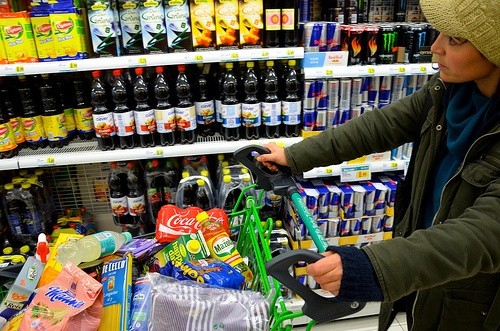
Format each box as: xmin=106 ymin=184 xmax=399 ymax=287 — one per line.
xmin=0 ymin=145 xmax=368 ymax=331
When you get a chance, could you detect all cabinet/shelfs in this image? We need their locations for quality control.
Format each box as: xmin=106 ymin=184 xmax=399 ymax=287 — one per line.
xmin=0 ymin=0 xmax=440 ymax=326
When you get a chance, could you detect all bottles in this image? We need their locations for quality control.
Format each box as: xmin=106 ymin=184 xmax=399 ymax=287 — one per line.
xmin=91 ymin=69 xmax=117 ymax=151
xmin=215 ymin=154 xmax=257 ymax=217
xmin=196 ymin=212 xmax=253 ymax=283
xmin=281 ymin=0 xmax=298 ymax=47
xmin=56 ymin=231 xmax=132 ymax=268
xmin=111 ymin=69 xmax=139 ymax=149
xmin=177 ymin=155 xmax=214 ymax=212
xmin=73 ymin=73 xmax=97 ymax=141
xmin=214 ymin=62 xmax=242 ymax=142
xmin=255 ymin=178 xmax=282 ymax=223
xmin=193 ymin=63 xmax=216 ymax=137
xmin=258 ymin=60 xmax=283 ymax=139
xmin=236 ymin=62 xmax=261 ymax=140
xmin=146 ymin=157 xmax=182 ymax=232
xmin=38 ymin=73 xmax=78 ymax=149
xmin=263 ymin=0 xmax=281 ymax=47
xmin=278 ymin=59 xmax=302 ymax=138
xmin=4 ymin=168 xmax=58 ymax=244
xmin=132 ymin=67 xmax=157 ymax=148
xmin=1 ymin=245 xmax=33 ymax=257
xmin=174 ymin=65 xmax=197 ymax=144
xmin=109 ymin=160 xmax=153 ymax=236
xmin=0 ymin=74 xmax=49 ymax=160
xmin=153 ymin=66 xmax=176 ymax=147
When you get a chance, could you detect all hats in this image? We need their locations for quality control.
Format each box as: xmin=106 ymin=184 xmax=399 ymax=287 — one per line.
xmin=419 ymin=0 xmax=500 ymax=68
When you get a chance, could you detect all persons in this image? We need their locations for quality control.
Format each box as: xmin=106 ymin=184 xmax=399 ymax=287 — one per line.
xmin=257 ymin=0 xmax=500 ymax=331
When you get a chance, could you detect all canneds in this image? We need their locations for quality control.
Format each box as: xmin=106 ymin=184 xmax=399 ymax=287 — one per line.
xmin=402 ymin=142 xmax=413 ymax=159
xmin=297 ymin=0 xmax=441 ymax=65
xmin=297 ymin=190 xmax=398 ymax=242
xmin=301 ymin=75 xmax=432 ymax=131
xmin=391 ymin=145 xmax=402 ymax=160
